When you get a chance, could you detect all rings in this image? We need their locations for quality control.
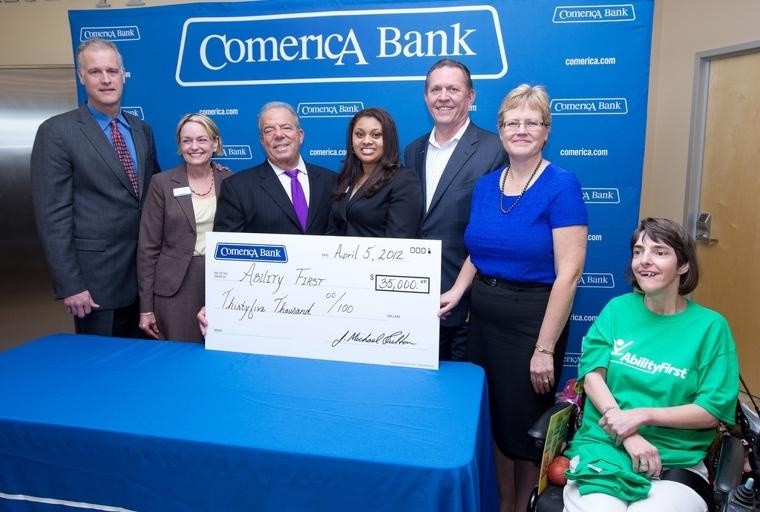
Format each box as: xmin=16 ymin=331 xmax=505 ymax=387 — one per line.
xmin=641 ymin=462 xmax=648 ymax=466
xmin=542 ymin=379 xmax=549 ymax=385
xmin=548 ymin=377 xmax=554 ymax=381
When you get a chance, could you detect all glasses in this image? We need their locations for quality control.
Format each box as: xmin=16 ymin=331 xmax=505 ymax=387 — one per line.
xmin=500 ymin=119 xmax=549 ymax=131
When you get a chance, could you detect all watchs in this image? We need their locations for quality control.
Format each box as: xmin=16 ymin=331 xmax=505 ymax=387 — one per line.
xmin=535 ymin=343 xmax=555 ymax=356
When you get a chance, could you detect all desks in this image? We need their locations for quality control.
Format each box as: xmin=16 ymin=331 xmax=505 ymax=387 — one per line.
xmin=0 ymin=332 xmax=501 ymax=511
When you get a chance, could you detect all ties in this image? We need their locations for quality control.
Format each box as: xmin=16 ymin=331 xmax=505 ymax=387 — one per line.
xmin=283 ymin=169 xmax=309 ymax=233
xmin=110 ymin=120 xmax=139 ymax=199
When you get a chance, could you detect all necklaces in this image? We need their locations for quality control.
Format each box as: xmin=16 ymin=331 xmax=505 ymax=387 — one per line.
xmin=500 ymin=158 xmax=543 ymax=214
xmin=189 ymin=167 xmax=213 ymax=196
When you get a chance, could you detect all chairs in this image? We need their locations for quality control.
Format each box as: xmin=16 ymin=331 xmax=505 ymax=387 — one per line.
xmin=527 ymin=377 xmax=745 ymax=511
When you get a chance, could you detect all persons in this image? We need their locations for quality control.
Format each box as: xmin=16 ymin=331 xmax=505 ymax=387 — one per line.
xmin=136 ymin=113 xmax=235 ymax=342
xmin=562 ymin=218 xmax=740 ymax=512
xmin=324 ymin=107 xmax=424 ymax=238
xmin=438 ymin=84 xmax=589 ymax=512
xmin=196 ymin=101 xmax=340 ymax=337
xmin=403 ymin=59 xmax=508 ymax=363
xmin=31 ymin=37 xmax=231 ymax=337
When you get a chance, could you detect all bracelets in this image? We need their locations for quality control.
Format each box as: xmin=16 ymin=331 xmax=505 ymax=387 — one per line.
xmin=602 ymin=406 xmax=615 ymax=414
xmin=139 ymin=313 xmax=152 ymax=315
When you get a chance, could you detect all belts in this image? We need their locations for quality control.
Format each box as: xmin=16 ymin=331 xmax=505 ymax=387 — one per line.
xmin=475 ymin=273 xmax=551 ymax=289
